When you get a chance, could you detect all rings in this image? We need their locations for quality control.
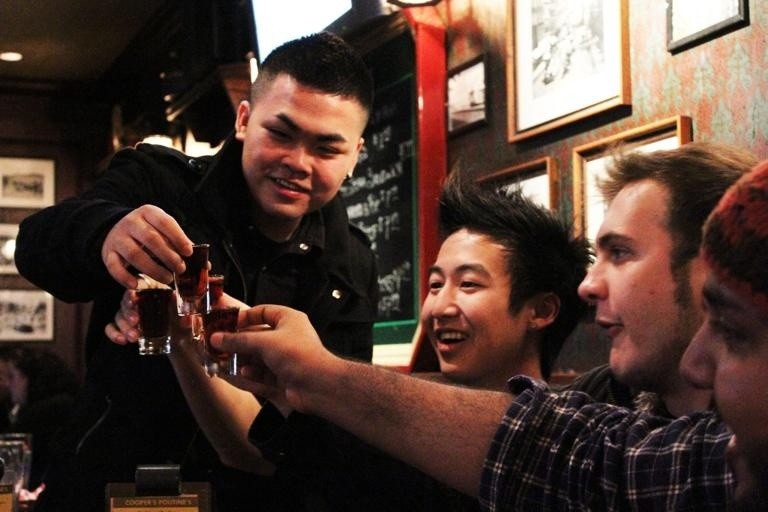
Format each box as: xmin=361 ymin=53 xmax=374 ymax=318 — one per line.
xmin=136 ymin=273 xmax=154 ymax=289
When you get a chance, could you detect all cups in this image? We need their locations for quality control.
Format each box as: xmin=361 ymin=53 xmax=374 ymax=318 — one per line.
xmin=134 ymin=244 xmax=239 ymax=378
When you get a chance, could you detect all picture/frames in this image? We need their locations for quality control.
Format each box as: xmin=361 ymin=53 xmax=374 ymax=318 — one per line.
xmin=0 ymin=219 xmax=23 ymax=279
xmin=469 ymin=153 xmax=560 ymax=220
xmin=0 ymin=285 xmax=57 ymax=344
xmin=663 ymin=2 xmax=749 ymax=55
xmin=570 ymin=112 xmax=697 ymax=273
xmin=444 ymin=50 xmax=493 ymax=137
xmin=0 ymin=154 xmax=57 ymax=211
xmin=502 ymin=0 xmax=632 ymax=146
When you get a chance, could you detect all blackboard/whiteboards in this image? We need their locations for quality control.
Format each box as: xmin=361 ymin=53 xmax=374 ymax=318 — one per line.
xmin=338 ymin=21 xmax=447 ymax=345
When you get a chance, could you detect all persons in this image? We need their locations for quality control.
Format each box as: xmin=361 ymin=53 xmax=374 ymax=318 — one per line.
xmin=6 ymin=346 xmax=75 ymax=432
xmin=11 ymin=30 xmax=383 ymax=512
xmin=101 ymin=156 xmax=595 ymax=512
